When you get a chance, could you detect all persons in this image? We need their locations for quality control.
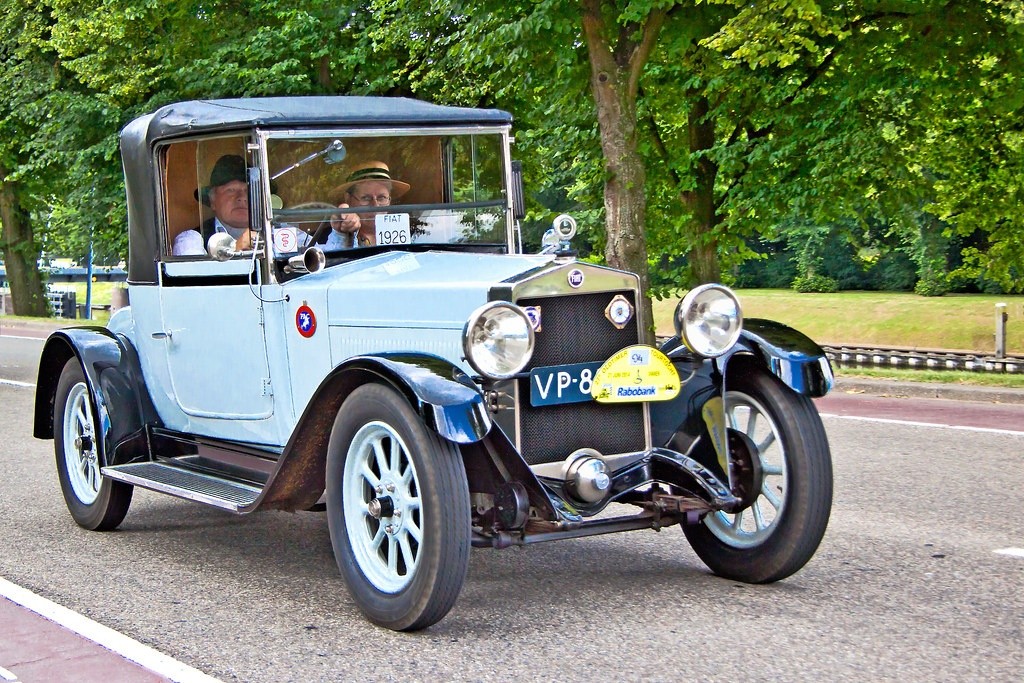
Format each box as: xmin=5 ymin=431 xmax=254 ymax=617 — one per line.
xmin=172 ymin=154 xmax=361 ymax=257
xmin=311 ymin=161 xmax=420 ymax=246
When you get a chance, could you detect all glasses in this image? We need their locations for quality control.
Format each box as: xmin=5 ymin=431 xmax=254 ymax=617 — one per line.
xmin=347 ymin=190 xmax=391 ymax=206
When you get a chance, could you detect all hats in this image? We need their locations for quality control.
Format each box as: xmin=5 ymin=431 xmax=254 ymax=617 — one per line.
xmin=328 ymin=160 xmax=411 ymax=207
xmin=193 ymin=154 xmax=278 ymax=207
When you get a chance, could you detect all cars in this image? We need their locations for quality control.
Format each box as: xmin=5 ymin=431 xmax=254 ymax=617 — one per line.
xmin=33 ymin=96 xmax=836 ymax=632
